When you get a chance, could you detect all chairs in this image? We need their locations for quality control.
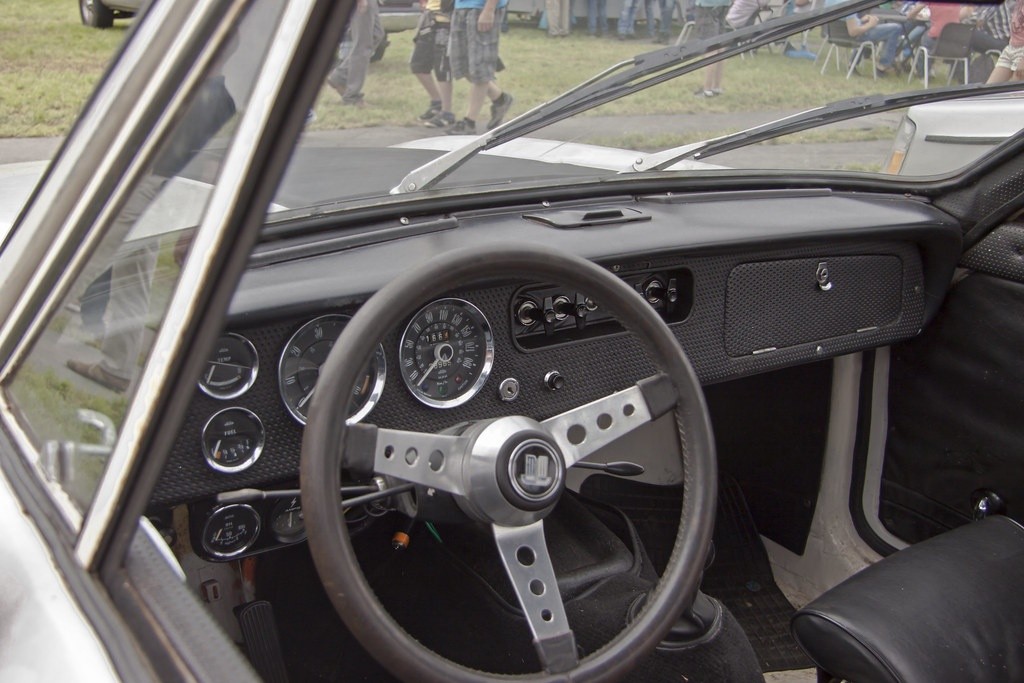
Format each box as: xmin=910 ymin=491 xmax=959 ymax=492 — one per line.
xmin=908 ymin=22 xmax=978 ymax=90
xmin=676 ymin=5 xmax=724 ymax=51
xmin=724 ymin=7 xmax=764 ymax=61
xmin=820 ymin=19 xmax=877 ymax=81
xmin=793 ymin=511 xmax=1024 ymax=683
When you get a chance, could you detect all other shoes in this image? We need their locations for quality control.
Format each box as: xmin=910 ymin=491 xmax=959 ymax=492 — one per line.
xmin=419 ymin=105 xmax=441 ymax=121
xmin=328 ymin=75 xmax=345 ymax=96
xmin=876 ymin=68 xmax=889 ymax=79
xmin=693 ymin=87 xmax=719 ymax=97
xmin=583 ymin=29 xmax=669 ymax=46
xmin=425 ymin=111 xmax=455 ymax=129
xmin=346 ymin=97 xmax=370 ymax=109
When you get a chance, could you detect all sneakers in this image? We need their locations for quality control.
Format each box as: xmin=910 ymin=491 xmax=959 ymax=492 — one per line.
xmin=447 ymin=118 xmax=475 ymax=135
xmin=488 ymin=93 xmax=512 ymax=128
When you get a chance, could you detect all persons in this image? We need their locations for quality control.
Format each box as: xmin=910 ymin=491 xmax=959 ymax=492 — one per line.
xmin=544 ymin=0 xmax=676 ymax=43
xmin=65 ymin=31 xmax=236 ymax=391
xmin=328 ymin=0 xmax=386 ymax=108
xmin=694 ymin=0 xmax=1024 ymax=97
xmin=410 ymin=0 xmax=514 ymax=135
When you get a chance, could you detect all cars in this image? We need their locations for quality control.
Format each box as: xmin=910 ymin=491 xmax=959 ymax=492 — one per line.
xmin=78 ymin=1 xmax=430 ymax=64
xmin=1 ymin=0 xmax=1024 ymax=683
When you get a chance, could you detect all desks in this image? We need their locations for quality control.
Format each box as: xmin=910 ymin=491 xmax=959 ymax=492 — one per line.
xmin=872 ymin=15 xmax=925 ymax=76
